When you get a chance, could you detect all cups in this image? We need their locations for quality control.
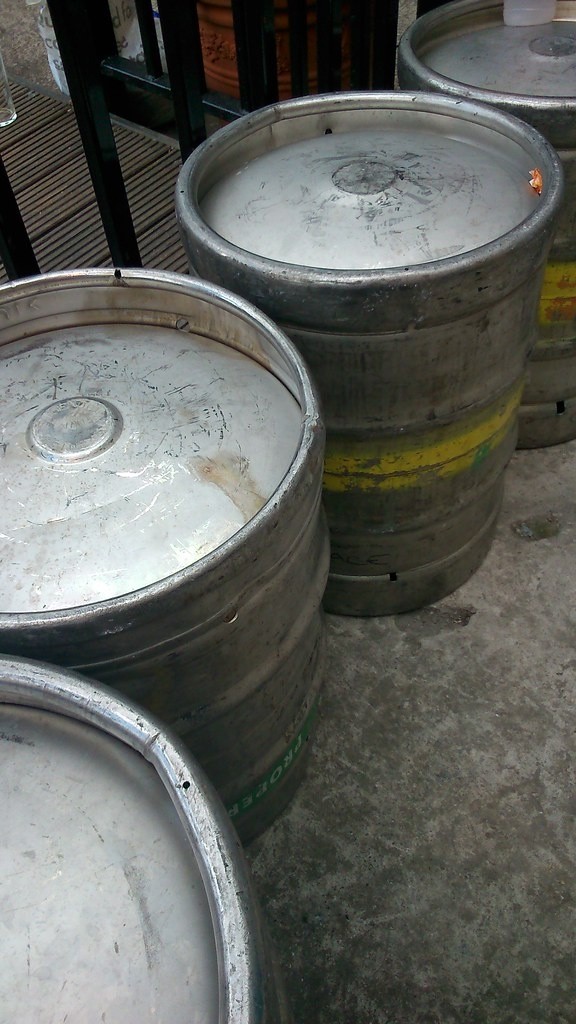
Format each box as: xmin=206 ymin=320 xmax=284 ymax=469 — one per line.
xmin=0 ymin=53 xmax=17 ymax=127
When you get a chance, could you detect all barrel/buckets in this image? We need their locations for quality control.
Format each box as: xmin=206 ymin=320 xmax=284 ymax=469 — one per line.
xmin=0 ymin=654 xmax=288 ymax=1024
xmin=399 ymin=0 xmax=576 ymax=449
xmin=0 ymin=270 xmax=330 ymax=841
xmin=176 ymin=91 xmax=561 ymax=616
